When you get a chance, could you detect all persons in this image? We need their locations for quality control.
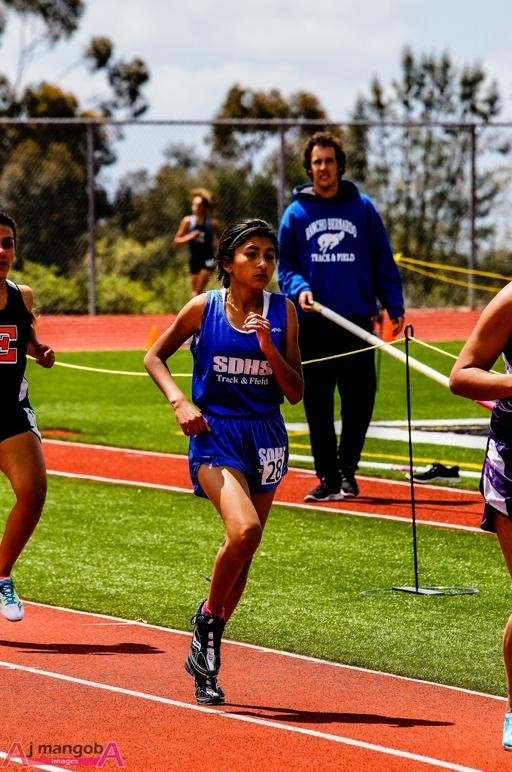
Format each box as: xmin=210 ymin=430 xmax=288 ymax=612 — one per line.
xmin=276 ymin=132 xmax=404 ymax=501
xmin=449 ymin=280 xmax=512 ymax=751
xmin=175 ymin=188 xmax=223 ymax=297
xmin=0 ymin=213 xmax=54 ymax=622
xmin=144 ymin=219 xmax=304 ymax=704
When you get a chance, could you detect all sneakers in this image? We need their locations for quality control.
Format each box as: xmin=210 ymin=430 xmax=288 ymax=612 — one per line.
xmin=340 ymin=471 xmax=359 ymax=497
xmin=184 ymin=656 xmax=225 ymax=705
xmin=502 ymin=712 xmax=512 ymax=748
xmin=304 ymin=478 xmax=344 ymax=502
xmin=189 ymin=598 xmax=226 ymax=677
xmin=412 ymin=463 xmax=461 ymax=484
xmin=1 ymin=577 xmax=25 ymax=622
